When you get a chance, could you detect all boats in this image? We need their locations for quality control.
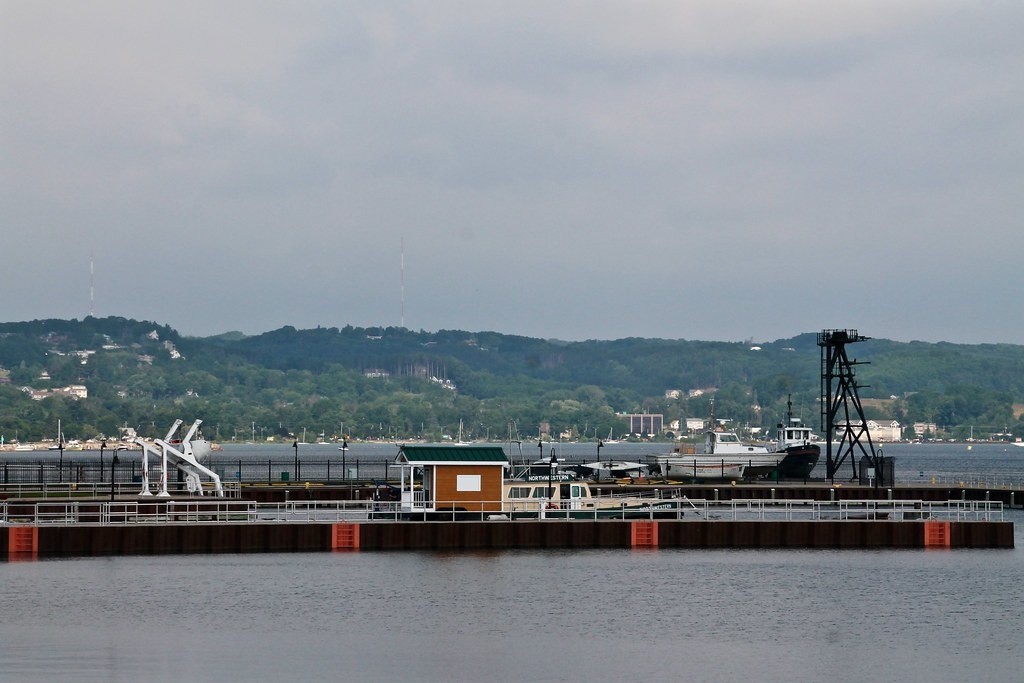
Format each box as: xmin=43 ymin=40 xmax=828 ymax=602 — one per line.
xmin=49 ymin=445 xmax=66 ymax=451
xmin=15 ymin=445 xmax=34 ymax=451
xmin=647 ymin=393 xmax=820 ymax=477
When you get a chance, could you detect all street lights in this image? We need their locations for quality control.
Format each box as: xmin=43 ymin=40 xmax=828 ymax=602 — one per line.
xmin=292 ymin=442 xmax=297 ymax=481
xmin=58 ymin=443 xmax=64 ymax=482
xmin=538 ymin=442 xmax=542 ymax=459
xmin=874 ymin=444 xmax=883 ymax=488
xmin=597 ymin=442 xmax=604 ymax=461
xmin=343 ymin=442 xmax=348 ymax=481
xmin=111 ymin=457 xmax=120 ymax=502
xmin=101 ymin=443 xmax=106 ymax=482
